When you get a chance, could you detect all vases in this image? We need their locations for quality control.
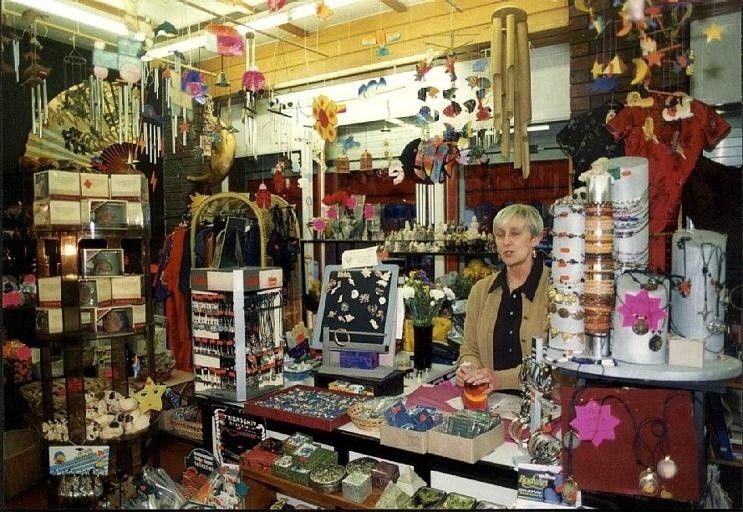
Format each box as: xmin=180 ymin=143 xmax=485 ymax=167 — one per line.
xmin=413 ymin=325 xmax=432 ymax=370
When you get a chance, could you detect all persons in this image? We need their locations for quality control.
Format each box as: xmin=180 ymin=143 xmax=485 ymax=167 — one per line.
xmin=452 ymin=202 xmax=555 ymax=396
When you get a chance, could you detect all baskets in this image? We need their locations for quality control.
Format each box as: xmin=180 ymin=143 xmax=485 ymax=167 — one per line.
xmin=3 ymin=376 xmax=114 ymax=500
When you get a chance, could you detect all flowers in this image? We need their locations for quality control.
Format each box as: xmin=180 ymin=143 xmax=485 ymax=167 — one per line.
xmin=402 ymin=270 xmax=456 ymax=328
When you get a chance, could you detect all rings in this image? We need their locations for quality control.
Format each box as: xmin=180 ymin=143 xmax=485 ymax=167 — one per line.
xmin=255 ymin=384 xmax=370 ymax=426
xmin=488 ymin=382 xmax=493 ymax=392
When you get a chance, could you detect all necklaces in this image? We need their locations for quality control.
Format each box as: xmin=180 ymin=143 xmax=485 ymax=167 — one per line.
xmin=506 ymin=280 xmax=517 ymax=291
xmin=615 ymin=287 xmax=674 ymax=336
xmin=705 ymin=243 xmax=734 ymax=337
xmin=562 ymin=389 xmax=707 ymax=506
xmin=646 ymin=276 xmax=668 ymax=352
xmin=610 ymin=185 xmax=650 ymax=271
xmin=698 ymin=240 xmax=715 ymax=325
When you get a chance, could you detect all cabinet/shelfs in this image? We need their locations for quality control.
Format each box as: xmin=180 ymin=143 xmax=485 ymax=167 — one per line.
xmin=28 ymin=222 xmax=162 ymax=512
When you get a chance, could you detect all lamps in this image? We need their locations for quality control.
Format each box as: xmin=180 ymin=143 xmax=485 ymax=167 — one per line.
xmin=145 ymin=1 xmax=357 ymax=58
xmin=0 ymin=0 xmax=130 ymax=36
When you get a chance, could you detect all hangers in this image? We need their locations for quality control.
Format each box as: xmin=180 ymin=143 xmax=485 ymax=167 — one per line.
xmin=603 ymin=89 xmax=620 ymax=112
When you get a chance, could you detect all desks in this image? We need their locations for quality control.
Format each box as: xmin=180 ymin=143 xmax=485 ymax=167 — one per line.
xmin=240 ymin=467 xmax=383 ymax=510
xmin=190 ymin=395 xmax=696 ymax=511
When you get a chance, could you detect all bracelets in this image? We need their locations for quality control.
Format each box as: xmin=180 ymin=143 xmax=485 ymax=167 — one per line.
xmin=579 ymin=196 xmax=622 ymax=338
xmin=332 ymin=326 xmax=352 ymax=347
xmin=506 ymin=409 xmax=561 ymax=468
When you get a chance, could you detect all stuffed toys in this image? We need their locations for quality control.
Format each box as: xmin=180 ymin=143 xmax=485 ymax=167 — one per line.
xmin=38 ymin=385 xmax=152 ymax=442
